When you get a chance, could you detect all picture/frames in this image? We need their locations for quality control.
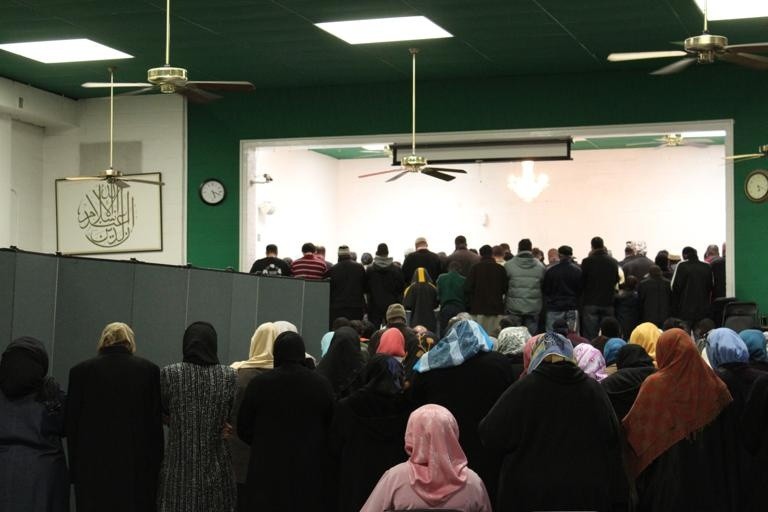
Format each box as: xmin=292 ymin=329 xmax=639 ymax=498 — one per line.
xmin=55 ymin=171 xmax=163 ymax=255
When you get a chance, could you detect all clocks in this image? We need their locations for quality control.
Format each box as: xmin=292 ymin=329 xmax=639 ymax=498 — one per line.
xmin=744 ymin=170 xmax=768 ymax=204
xmin=200 ymin=178 xmax=226 ymax=206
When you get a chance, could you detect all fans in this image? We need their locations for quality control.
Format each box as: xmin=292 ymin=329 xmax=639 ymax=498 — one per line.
xmin=66 ymin=67 xmax=168 ymax=189
xmin=81 ymin=0 xmax=254 ymax=104
xmin=604 ymin=0 xmax=767 ymax=77
xmin=359 ymin=44 xmax=467 ymax=185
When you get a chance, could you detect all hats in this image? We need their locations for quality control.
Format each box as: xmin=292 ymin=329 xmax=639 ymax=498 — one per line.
xmin=386 ymin=304 xmax=407 ymax=321
xmin=558 ymin=246 xmax=572 ymax=255
xmin=416 ymin=237 xmax=426 ymax=243
xmin=339 ymin=246 xmax=350 ymax=255
xmin=667 ymin=253 xmax=681 ymax=260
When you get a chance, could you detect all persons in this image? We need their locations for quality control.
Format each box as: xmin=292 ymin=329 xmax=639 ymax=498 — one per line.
xmin=356 ymin=401 xmax=495 ymax=511
xmin=328 ymin=236 xmax=725 ymax=335
xmin=238 ymin=330 xmax=344 ymax=510
xmin=479 ymin=330 xmax=630 ymax=510
xmin=229 ymin=304 xmax=768 ymax=483
xmin=154 ymin=321 xmax=233 ymax=512
xmin=0 ymin=332 xmax=71 ymax=511
xmin=64 ymin=318 xmax=164 ymax=511
xmin=704 ymin=321 xmax=768 ymax=510
xmin=333 ymin=355 xmax=414 ymax=511
xmin=623 ymin=327 xmax=737 ymax=509
xmin=249 ymin=242 xmax=333 ymax=283
